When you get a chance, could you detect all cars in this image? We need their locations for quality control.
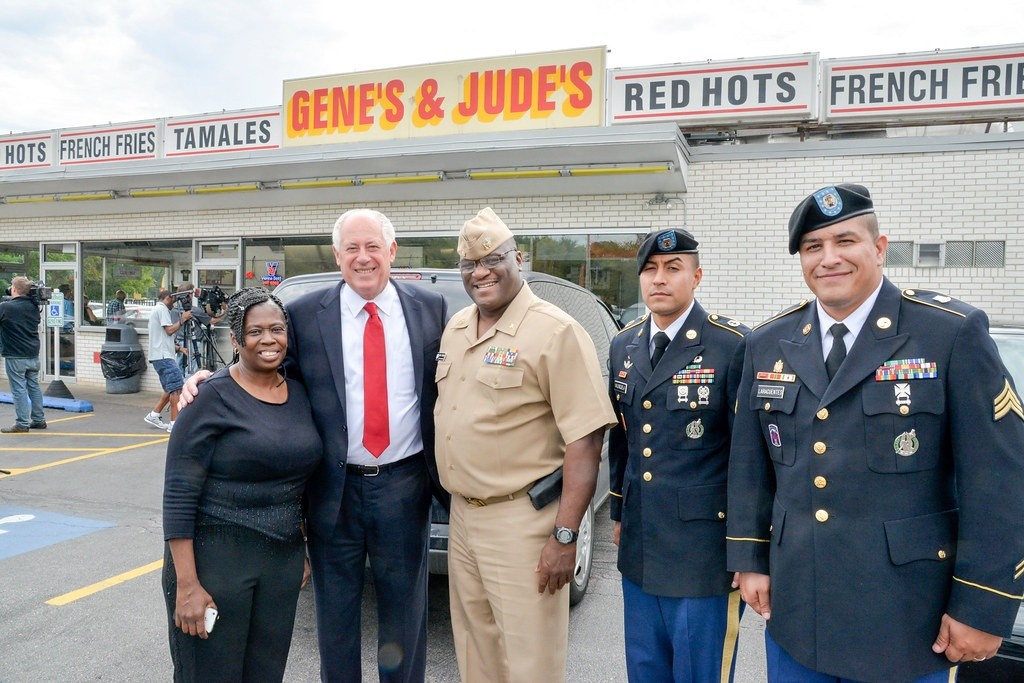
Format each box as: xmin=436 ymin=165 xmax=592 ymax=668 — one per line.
xmin=954 ymin=324 xmax=1024 ymax=683
xmin=87 ymin=303 xmax=152 ymax=318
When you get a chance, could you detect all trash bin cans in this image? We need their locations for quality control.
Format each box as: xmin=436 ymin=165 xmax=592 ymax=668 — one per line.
xmin=101 ymin=324 xmax=142 ymax=393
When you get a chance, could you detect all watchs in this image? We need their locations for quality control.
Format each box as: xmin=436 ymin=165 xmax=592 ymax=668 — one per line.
xmin=552 ymin=525 xmax=579 ymax=544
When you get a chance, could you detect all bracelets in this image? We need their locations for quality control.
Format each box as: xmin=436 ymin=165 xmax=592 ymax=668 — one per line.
xmin=178 ymin=346 xmax=181 ymax=351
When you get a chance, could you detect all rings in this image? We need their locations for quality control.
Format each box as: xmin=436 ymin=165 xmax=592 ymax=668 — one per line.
xmin=974 ymin=657 xmax=985 ymax=662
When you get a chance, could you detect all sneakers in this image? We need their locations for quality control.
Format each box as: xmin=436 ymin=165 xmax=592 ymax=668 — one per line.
xmin=143 ymin=413 xmax=169 ymax=429
xmin=166 ymin=423 xmax=174 ymax=433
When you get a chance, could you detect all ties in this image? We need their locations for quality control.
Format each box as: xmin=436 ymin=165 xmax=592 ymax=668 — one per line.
xmin=826 ymin=323 xmax=850 ymax=381
xmin=651 ymin=332 xmax=671 ymax=373
xmin=364 ymin=302 xmax=390 ymax=458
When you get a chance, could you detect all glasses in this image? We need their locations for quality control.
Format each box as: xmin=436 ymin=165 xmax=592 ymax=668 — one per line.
xmin=455 ymin=248 xmax=515 ymax=274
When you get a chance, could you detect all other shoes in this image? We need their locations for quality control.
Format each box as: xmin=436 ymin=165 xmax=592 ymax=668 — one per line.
xmin=1 ymin=423 xmax=29 ymax=433
xmin=29 ymin=421 xmax=47 ymax=429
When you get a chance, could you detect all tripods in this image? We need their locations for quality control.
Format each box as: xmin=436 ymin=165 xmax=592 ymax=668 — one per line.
xmin=182 ymin=314 xmax=226 ymax=377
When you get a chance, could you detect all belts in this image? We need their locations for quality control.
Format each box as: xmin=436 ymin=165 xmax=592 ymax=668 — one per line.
xmin=464 ymin=483 xmax=534 ymax=507
xmin=346 ymin=452 xmax=423 ymax=478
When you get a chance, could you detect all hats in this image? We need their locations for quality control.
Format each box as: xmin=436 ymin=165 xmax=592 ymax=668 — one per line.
xmin=637 ymin=227 xmax=700 ymax=275
xmin=788 ymin=183 xmax=875 ymax=254
xmin=455 ymin=207 xmax=513 ymax=261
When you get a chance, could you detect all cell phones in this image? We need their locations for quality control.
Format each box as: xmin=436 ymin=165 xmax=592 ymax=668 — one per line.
xmin=171 ymin=608 xmax=220 ymax=633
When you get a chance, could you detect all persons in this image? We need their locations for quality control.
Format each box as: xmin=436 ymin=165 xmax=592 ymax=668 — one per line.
xmin=177 ymin=208 xmax=447 ymax=683
xmin=143 ymin=282 xmax=228 ymax=433
xmin=433 ymin=206 xmax=618 ymax=683
xmin=0 ymin=277 xmax=46 ymax=434
xmin=162 ymin=286 xmax=319 ymax=683
xmin=53 ymin=283 xmax=126 ymax=366
xmin=606 ymin=228 xmax=757 ymax=683
xmin=724 ymin=184 xmax=1024 ymax=683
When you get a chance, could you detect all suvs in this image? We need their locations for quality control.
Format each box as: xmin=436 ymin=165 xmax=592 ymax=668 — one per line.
xmin=273 ymin=266 xmax=626 ymax=606
